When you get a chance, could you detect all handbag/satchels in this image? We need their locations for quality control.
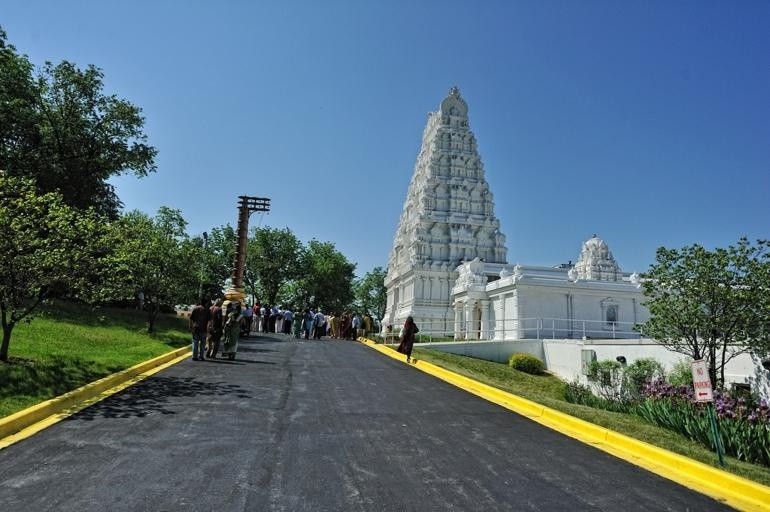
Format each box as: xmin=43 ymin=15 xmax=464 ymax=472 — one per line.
xmin=312 ymin=314 xmax=319 ymax=327
xmin=413 ymin=323 xmax=419 ymax=334
xmin=208 ymin=318 xmax=223 ymax=340
xmin=398 ymin=329 xmax=404 ymax=342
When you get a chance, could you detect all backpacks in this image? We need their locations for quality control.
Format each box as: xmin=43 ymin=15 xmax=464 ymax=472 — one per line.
xmin=191 ymin=308 xmax=207 ymax=336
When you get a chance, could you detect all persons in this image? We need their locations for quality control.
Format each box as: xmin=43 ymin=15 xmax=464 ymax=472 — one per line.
xmin=189 ymin=300 xmax=373 ymax=361
xmin=397 ymin=315 xmax=419 ymax=363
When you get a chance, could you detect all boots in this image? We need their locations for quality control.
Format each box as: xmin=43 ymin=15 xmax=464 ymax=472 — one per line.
xmin=192 ymin=352 xmax=205 ymax=361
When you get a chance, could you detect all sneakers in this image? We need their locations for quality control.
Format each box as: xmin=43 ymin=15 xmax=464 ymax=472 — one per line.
xmin=206 ymin=352 xmax=217 ymax=360
xmin=227 ymin=357 xmax=236 ymax=361
xmin=407 ymin=358 xmax=411 ymax=363
